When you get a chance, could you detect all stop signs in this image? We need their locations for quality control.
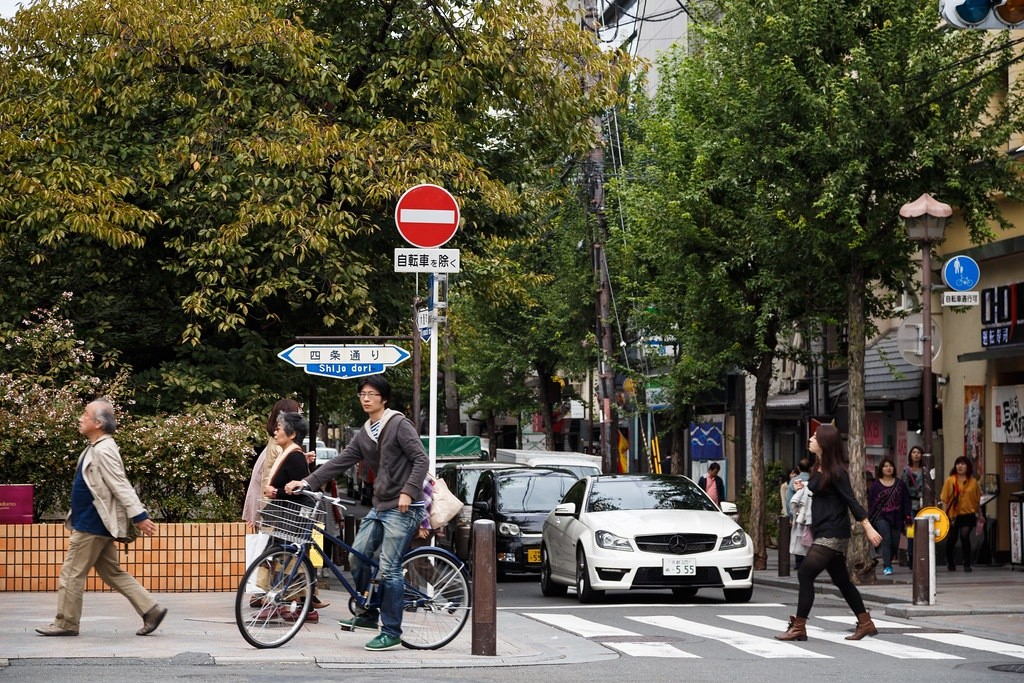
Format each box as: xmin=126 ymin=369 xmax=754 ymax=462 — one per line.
xmin=394 ymin=183 xmax=461 ymax=248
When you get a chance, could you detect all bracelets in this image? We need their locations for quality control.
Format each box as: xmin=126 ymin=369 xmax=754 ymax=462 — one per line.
xmin=860 ymin=519 xmax=869 ymax=525
xmin=273 ymin=488 xmax=278 ymax=497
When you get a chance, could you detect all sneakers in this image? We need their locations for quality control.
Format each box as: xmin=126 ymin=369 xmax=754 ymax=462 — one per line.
xmin=251 ymin=605 xmax=290 ymax=619
xmin=364 ymin=633 xmax=402 ymax=650
xmin=282 ymin=608 xmax=319 ymax=624
xmin=338 ymin=614 xmax=379 ymax=631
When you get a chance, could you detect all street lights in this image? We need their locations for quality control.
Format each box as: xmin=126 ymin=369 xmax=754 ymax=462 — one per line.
xmin=898 ymin=191 xmax=955 ymax=598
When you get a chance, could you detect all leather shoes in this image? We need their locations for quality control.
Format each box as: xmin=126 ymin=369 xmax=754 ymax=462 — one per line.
xmin=136 ymin=605 xmax=167 ymax=635
xmin=34 ymin=624 xmax=79 ymax=636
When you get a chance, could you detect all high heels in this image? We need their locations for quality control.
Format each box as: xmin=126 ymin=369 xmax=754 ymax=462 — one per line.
xmin=844 ymin=612 xmax=877 ymax=640
xmin=774 ymin=616 xmax=807 ymax=641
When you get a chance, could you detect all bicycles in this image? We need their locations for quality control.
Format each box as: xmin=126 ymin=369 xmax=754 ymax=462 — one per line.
xmin=235 ymin=486 xmax=473 ymax=651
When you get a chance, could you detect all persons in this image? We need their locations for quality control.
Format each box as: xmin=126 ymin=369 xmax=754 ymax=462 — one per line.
xmin=698 ymin=462 xmax=725 ymax=511
xmin=242 ymin=399 xmax=330 ymax=624
xmin=774 ymin=425 xmax=883 ymax=641
xmin=404 ymin=472 xmax=464 ymax=614
xmin=34 ymin=398 xmax=168 ymax=636
xmin=284 ymin=374 xmax=430 ymax=651
xmin=779 ymin=446 xmax=985 ymax=574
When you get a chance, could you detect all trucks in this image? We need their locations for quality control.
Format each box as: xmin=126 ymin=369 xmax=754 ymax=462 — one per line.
xmin=421 ymin=435 xmax=489 ymax=475
xmin=342 ymin=426 xmax=373 ymax=506
xmin=496 ymin=448 xmax=606 ymax=480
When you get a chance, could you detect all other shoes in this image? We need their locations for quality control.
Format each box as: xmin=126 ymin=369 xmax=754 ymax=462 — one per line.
xmin=947 ymin=559 xmax=956 ymax=572
xmin=883 ymin=567 xmax=894 ymax=575
xmin=963 ymin=563 xmax=972 ymax=572
xmin=310 ymin=602 xmax=330 ymax=609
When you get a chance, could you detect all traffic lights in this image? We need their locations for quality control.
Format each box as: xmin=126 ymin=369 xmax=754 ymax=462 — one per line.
xmin=941 ymin=0 xmax=1024 ymax=29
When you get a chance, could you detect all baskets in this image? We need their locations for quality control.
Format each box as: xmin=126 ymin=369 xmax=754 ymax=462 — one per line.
xmin=256 ymin=498 xmax=328 ymax=543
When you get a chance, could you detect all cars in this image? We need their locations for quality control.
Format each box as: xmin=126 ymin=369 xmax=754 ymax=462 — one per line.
xmin=471 ymin=469 xmax=582 ymax=580
xmin=542 ymin=474 xmax=755 ymax=603
xmin=302 ymin=438 xmax=338 ymax=472
xmin=437 ymin=462 xmax=530 ymax=574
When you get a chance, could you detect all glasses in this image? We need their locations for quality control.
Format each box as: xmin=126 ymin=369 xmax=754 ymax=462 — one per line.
xmin=357 ymin=393 xmax=381 ymax=397
xmin=275 ymin=428 xmax=291 ymax=433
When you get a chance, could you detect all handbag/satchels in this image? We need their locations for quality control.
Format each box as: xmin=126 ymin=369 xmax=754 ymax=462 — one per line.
xmin=114 ymin=514 xmax=140 ymax=544
xmin=428 ymin=473 xmax=464 ymax=529
